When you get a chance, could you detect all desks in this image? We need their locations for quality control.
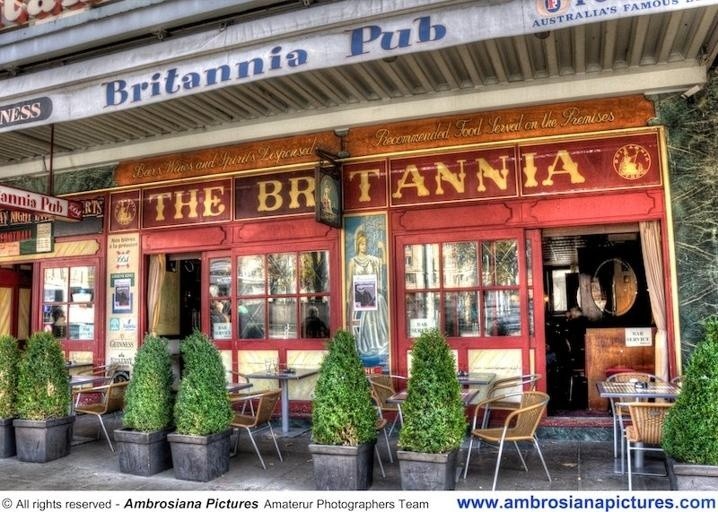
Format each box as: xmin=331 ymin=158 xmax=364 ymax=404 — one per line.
xmin=455 ymin=372 xmax=496 ymax=384
xmin=245 ymin=366 xmax=321 ymax=439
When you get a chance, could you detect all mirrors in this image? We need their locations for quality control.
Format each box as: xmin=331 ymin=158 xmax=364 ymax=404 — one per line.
xmin=591 ymin=258 xmax=638 ymax=316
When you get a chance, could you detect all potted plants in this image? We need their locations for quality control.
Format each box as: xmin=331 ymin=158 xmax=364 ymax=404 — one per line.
xmin=13 ymin=329 xmax=75 ymax=462
xmin=166 ymin=328 xmax=234 ymax=483
xmin=662 ymin=313 xmax=718 ymax=491
xmin=0 ymin=333 xmax=20 ymax=458
xmin=397 ymin=327 xmax=467 ymax=489
xmin=114 ymin=333 xmax=175 ymax=477
xmin=308 ymin=329 xmax=379 ymax=490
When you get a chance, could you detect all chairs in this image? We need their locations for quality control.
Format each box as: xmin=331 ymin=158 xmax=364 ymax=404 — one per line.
xmin=225 ymin=370 xmax=283 ymax=470
xmin=626 ymin=401 xmax=673 ymax=491
xmin=478 ymin=373 xmax=542 ymax=449
xmin=463 ymin=391 xmax=552 ymax=490
xmin=66 ymin=362 xmax=130 ymax=454
xmin=607 ymin=372 xmax=661 ymax=459
xmin=562 ymin=336 xmax=585 ymax=401
xmin=368 ymin=394 xmax=393 ymax=477
xmin=367 ymin=373 xmax=410 ymax=434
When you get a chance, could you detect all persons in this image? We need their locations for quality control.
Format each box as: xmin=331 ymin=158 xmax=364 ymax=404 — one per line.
xmin=563 ymin=305 xmax=590 ymax=362
xmin=45 ymin=307 xmax=67 ymax=339
xmin=344 ymin=225 xmax=390 ymax=372
xmin=301 ymin=305 xmax=327 ymax=338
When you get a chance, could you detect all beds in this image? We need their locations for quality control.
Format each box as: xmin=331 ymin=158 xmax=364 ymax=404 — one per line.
xmin=596 ymin=381 xmax=681 ymax=474
